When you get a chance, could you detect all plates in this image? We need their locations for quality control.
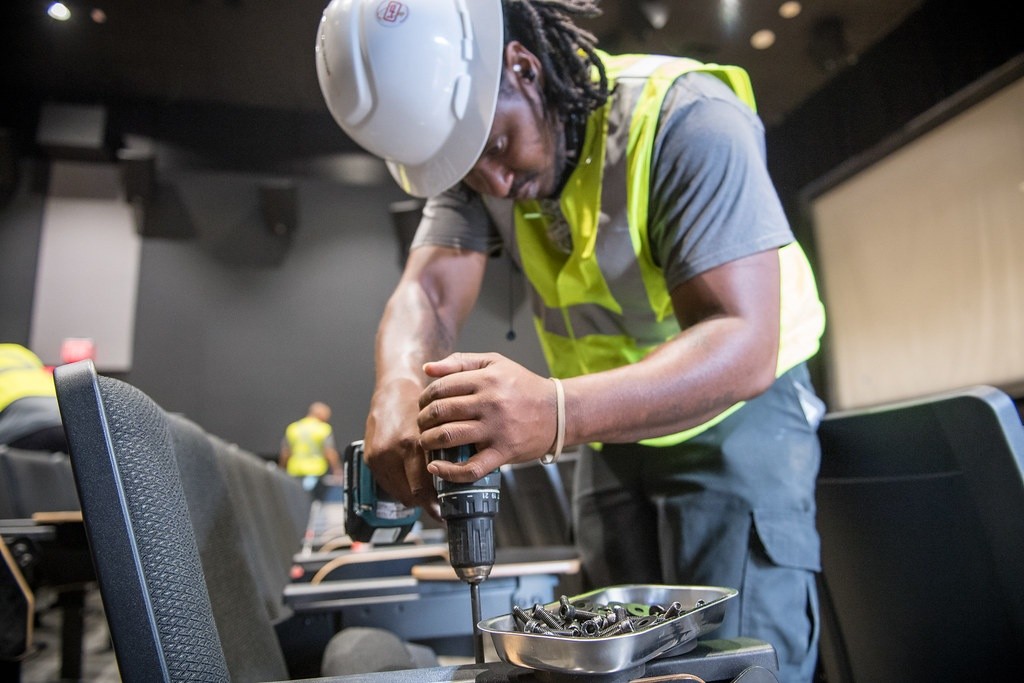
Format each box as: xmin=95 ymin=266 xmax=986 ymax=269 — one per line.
xmin=475 ymin=585 xmax=741 ymax=675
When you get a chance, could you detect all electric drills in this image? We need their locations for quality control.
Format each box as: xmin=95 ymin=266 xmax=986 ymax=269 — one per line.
xmin=342 ymin=428 xmax=502 ymax=665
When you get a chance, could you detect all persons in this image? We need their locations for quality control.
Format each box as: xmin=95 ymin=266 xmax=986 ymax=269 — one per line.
xmin=280 ymin=401 xmax=344 ymax=483
xmin=312 ymin=0 xmax=825 ymax=683
xmin=1 ymin=341 xmax=71 ymax=458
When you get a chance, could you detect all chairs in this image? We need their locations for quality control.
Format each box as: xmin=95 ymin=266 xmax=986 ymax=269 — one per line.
xmin=814 ymin=384 xmax=1024 ymax=683
xmin=0 ymin=355 xmax=787 ymax=683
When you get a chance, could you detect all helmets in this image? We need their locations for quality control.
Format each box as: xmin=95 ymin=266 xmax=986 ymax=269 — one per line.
xmin=315 ymin=0 xmax=504 ymax=199
